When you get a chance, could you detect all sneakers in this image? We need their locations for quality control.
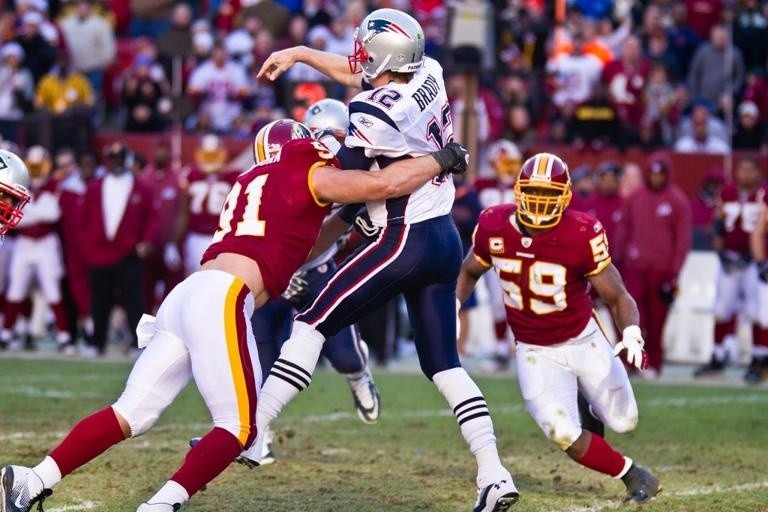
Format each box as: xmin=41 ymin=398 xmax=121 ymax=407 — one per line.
xmin=621 ymin=463 xmax=664 ymax=505
xmin=190 ymin=431 xmax=275 ymax=468
xmin=135 ymin=501 xmax=182 ymax=512
xmin=743 ymin=354 xmax=768 ymax=382
xmin=578 ymin=391 xmax=604 ymax=439
xmin=2 ymin=465 xmax=52 ymax=512
xmin=473 ymin=465 xmax=519 ymax=512
xmin=695 ymin=352 xmax=731 ymax=374
xmin=348 ymin=367 xmax=381 ymax=424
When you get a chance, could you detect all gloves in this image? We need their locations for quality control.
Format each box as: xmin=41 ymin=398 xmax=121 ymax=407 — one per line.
xmin=456 ymin=296 xmax=463 ymax=341
xmin=431 ymin=143 xmax=470 ymax=178
xmin=612 ymin=324 xmax=649 ymax=369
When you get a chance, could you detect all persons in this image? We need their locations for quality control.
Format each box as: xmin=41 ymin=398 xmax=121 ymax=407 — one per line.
xmin=692 ymin=158 xmax=768 ymax=386
xmin=59 ymin=144 xmax=104 ymax=358
xmin=139 ymin=143 xmax=186 ymax=316
xmin=444 ymin=56 xmax=502 ymax=150
xmin=165 ymin=135 xmax=242 ymax=277
xmin=566 ymin=166 xmax=596 ymax=216
xmin=265 ymin=12 xmax=310 ymax=54
xmin=310 ymin=16 xmax=362 ymax=100
xmin=501 ymin=0 xmax=768 ymax=152
xmin=188 ymin=8 xmax=519 ymax=512
xmin=223 ymin=13 xmax=263 ymax=60
xmin=0 ymin=146 xmax=71 ymax=351
xmin=189 ymin=99 xmax=383 ymax=468
xmin=362 ymin=0 xmax=492 ymax=46
xmin=0 ymin=148 xmax=30 ymax=243
xmin=455 ymin=152 xmax=664 ymax=505
xmin=189 ymin=43 xmax=249 ymax=134
xmin=77 ymin=141 xmax=147 ymax=356
xmin=609 ymin=163 xmax=642 ymax=268
xmin=585 ymin=161 xmax=622 ymax=305
xmin=0 ymin=0 xmax=183 ymax=140
xmin=241 ymin=28 xmax=273 ymax=98
xmin=0 ymin=120 xmax=470 ymax=512
xmin=614 ymin=157 xmax=692 ymax=380
xmin=469 ymin=140 xmax=520 ymax=374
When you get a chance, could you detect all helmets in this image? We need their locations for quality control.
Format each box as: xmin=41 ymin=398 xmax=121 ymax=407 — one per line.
xmin=513 ymin=152 xmax=572 ymax=228
xmin=252 ymin=119 xmax=315 ymax=164
xmin=348 ymin=9 xmax=426 ymax=79
xmin=0 ymin=149 xmax=32 ymax=236
xmin=299 ymin=99 xmax=349 ymax=135
